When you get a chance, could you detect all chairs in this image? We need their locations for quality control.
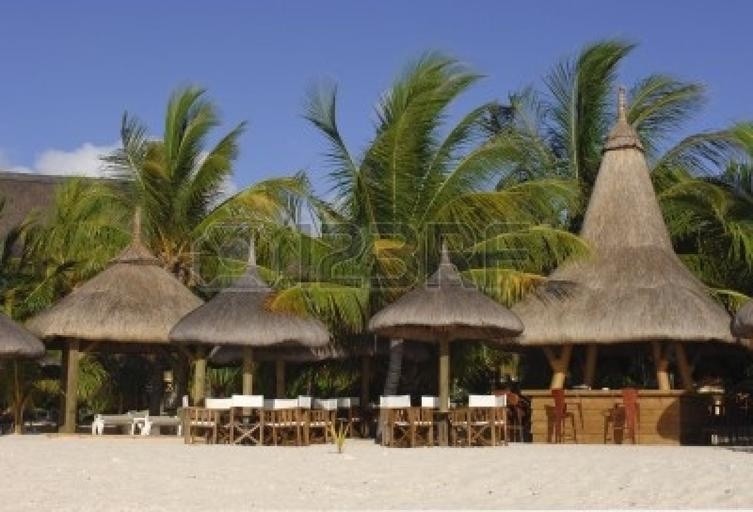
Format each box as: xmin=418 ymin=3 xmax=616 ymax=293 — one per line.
xmin=548 ymin=387 xmax=577 ymax=444
xmin=607 ymin=384 xmax=640 ymax=444
xmin=83 ymin=380 xmax=531 ymax=452
